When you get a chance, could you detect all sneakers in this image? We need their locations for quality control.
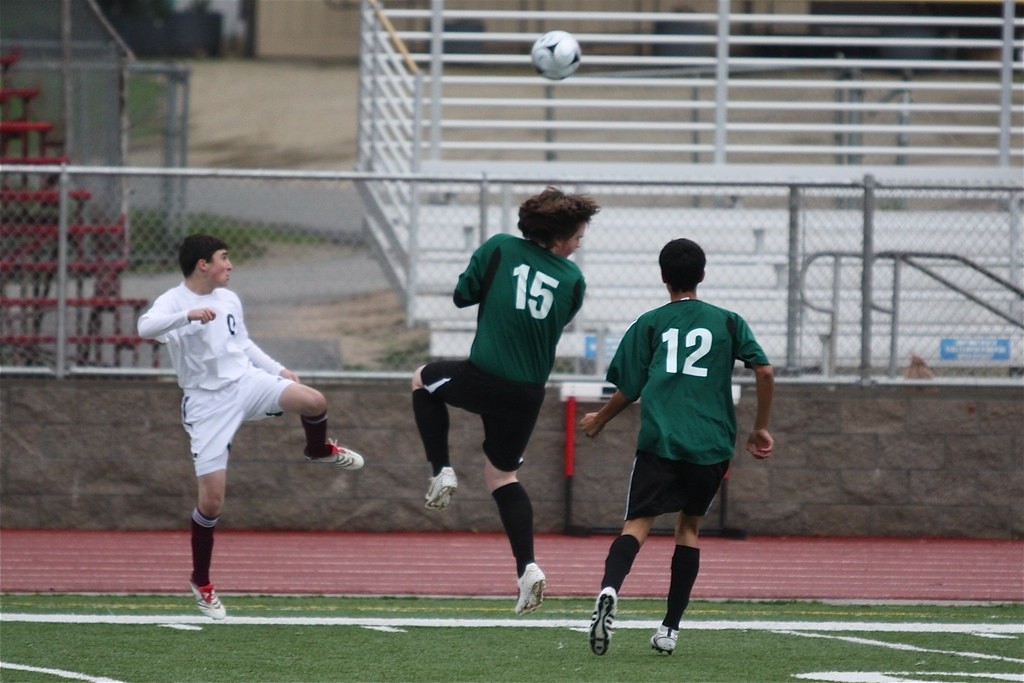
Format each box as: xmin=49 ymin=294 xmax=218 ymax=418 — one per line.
xmin=589 ymin=586 xmax=617 ymax=655
xmin=650 ymin=623 xmax=680 ymax=655
xmin=190 ymin=575 xmax=226 ymax=620
xmin=304 ymin=438 xmax=365 ymax=470
xmin=515 ymin=563 xmax=545 ymax=618
xmin=424 ymin=467 xmax=458 ymax=511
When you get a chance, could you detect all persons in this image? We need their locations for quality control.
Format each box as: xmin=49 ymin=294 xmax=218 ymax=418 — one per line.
xmin=576 ymin=238 xmax=775 ymax=656
xmin=137 ymin=235 xmax=364 ymax=619
xmin=412 ymin=188 xmax=601 ymax=616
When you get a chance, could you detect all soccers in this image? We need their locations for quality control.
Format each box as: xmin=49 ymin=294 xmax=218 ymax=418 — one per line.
xmin=531 ymin=31 xmax=582 ymax=80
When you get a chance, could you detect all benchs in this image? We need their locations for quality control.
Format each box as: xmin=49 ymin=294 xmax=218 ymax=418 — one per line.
xmin=0 ymin=47 xmax=164 ymax=366
xmin=414 ymin=161 xmax=1024 ymax=371
